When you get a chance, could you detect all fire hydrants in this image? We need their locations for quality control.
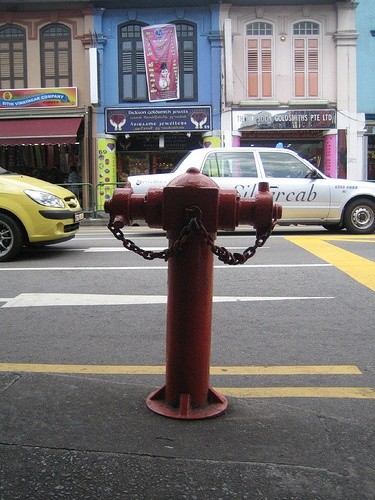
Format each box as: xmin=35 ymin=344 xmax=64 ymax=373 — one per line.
xmin=103 ymin=166 xmax=284 ymax=423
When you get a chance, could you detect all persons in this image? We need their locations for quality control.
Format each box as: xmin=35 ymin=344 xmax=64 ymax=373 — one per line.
xmin=48 ymin=166 xmax=64 ymax=183
xmin=32 ymin=170 xmax=43 ymax=178
xmin=68 ymin=166 xmax=82 ymax=193
xmin=120 ymin=170 xmax=129 ymax=181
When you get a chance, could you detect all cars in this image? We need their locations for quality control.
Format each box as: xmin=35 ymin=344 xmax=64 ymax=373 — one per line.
xmin=124 ymin=147 xmax=375 ymax=233
xmin=0 ymin=167 xmax=85 ymax=262
